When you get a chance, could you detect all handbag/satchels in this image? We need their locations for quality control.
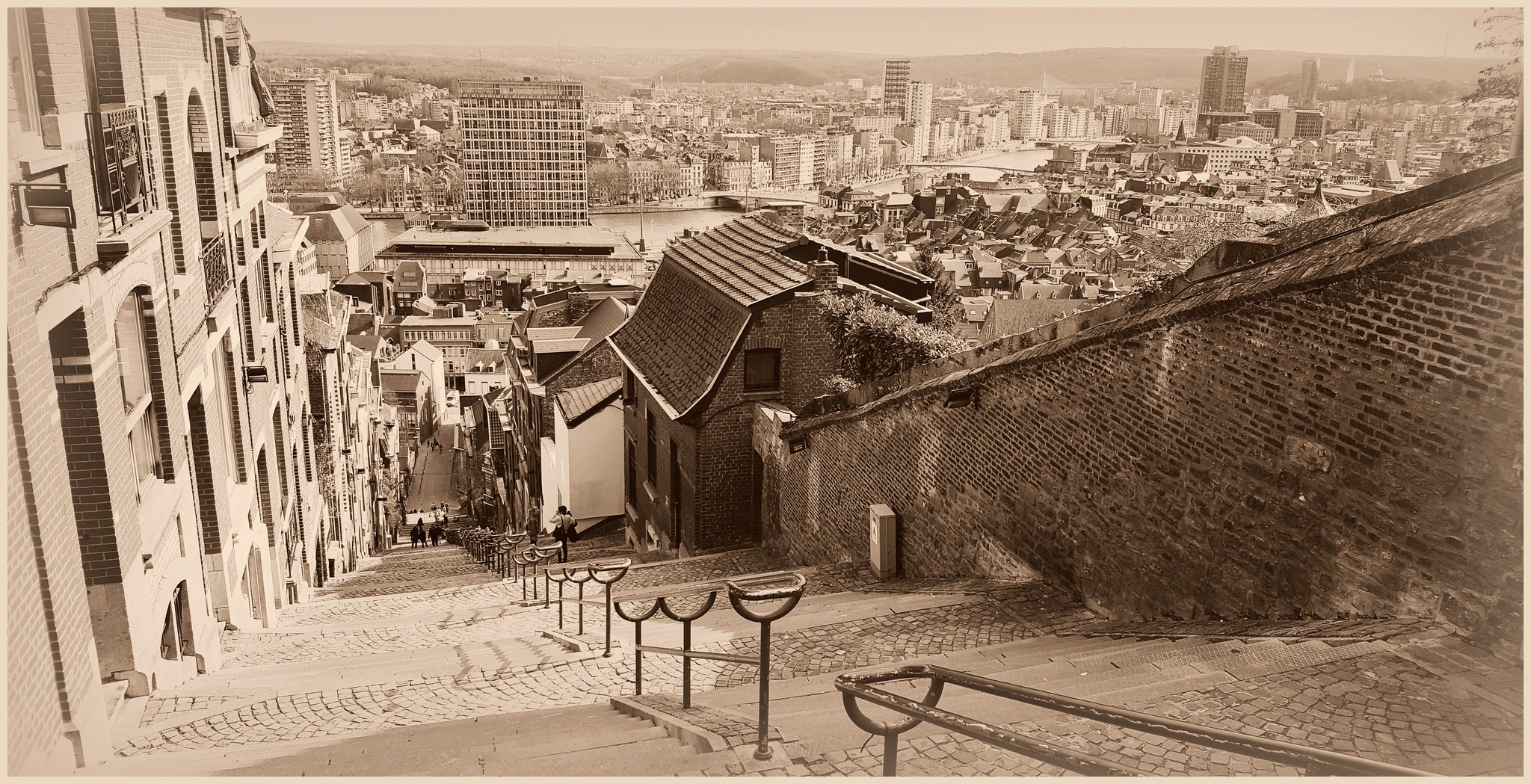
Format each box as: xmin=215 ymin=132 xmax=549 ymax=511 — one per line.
xmin=553 ymin=524 xmax=566 ymax=539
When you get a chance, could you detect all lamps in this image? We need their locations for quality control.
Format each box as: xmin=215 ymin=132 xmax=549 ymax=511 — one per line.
xmin=527 ymin=470 xmax=536 ymax=475
xmin=367 ymin=480 xmax=376 ymax=485
xmin=623 ymin=396 xmax=637 ymax=408
xmin=942 ymin=385 xmax=979 ymax=409
xmin=13 ymin=181 xmax=77 ymax=229
xmin=355 ymin=468 xmax=365 ymax=475
xmin=342 ymin=449 xmax=351 ymax=455
xmin=382 ymin=497 xmax=387 ymax=501
xmin=243 ymin=365 xmax=269 ymax=382
xmin=788 ymin=432 xmax=808 ymax=454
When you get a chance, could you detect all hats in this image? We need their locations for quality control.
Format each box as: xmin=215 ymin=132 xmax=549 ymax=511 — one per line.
xmin=531 ymin=506 xmax=540 ymax=512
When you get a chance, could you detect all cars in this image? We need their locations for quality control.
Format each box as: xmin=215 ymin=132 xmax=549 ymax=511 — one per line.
xmin=633 ymin=241 xmax=640 ymax=245
xmin=863 ymin=166 xmax=891 ymax=179
xmin=589 ymin=200 xmax=634 ymax=206
xmin=646 ymin=194 xmax=683 ymax=201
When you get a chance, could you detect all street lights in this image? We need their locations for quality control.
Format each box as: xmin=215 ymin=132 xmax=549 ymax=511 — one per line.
xmin=610 ymin=221 xmax=613 ymax=229
xmin=700 ymin=217 xmax=703 ymax=230
xmin=651 ymin=219 xmax=655 ymax=243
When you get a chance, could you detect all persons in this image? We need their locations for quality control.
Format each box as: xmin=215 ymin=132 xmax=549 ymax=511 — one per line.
xmin=528 ymin=506 xmax=540 ymax=560
xmin=427 ymin=436 xmax=443 ymax=454
xmin=550 ymin=506 xmax=575 ymax=564
xmin=407 ymin=501 xmax=460 ymax=549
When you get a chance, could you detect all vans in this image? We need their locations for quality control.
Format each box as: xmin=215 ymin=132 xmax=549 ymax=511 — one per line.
xmin=446 ymin=390 xmax=460 ymax=406
